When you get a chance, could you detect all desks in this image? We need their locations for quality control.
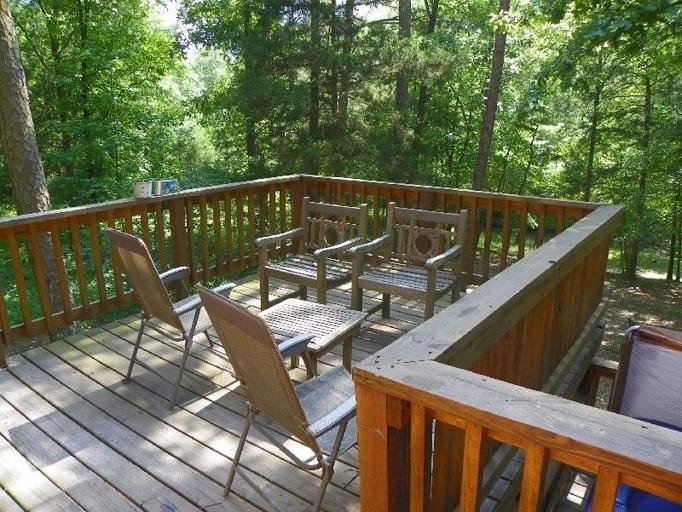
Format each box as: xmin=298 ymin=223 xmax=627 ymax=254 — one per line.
xmin=252 ymin=299 xmax=368 ymax=380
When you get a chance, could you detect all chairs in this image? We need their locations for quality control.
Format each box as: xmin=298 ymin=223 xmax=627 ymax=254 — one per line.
xmin=195 ymin=283 xmax=356 ymax=512
xmin=583 ymin=326 xmax=682 ymax=512
xmin=257 ymin=198 xmax=368 ymax=310
xmin=349 ymin=202 xmax=468 ymax=336
xmin=106 ymin=227 xmax=237 ymax=409
